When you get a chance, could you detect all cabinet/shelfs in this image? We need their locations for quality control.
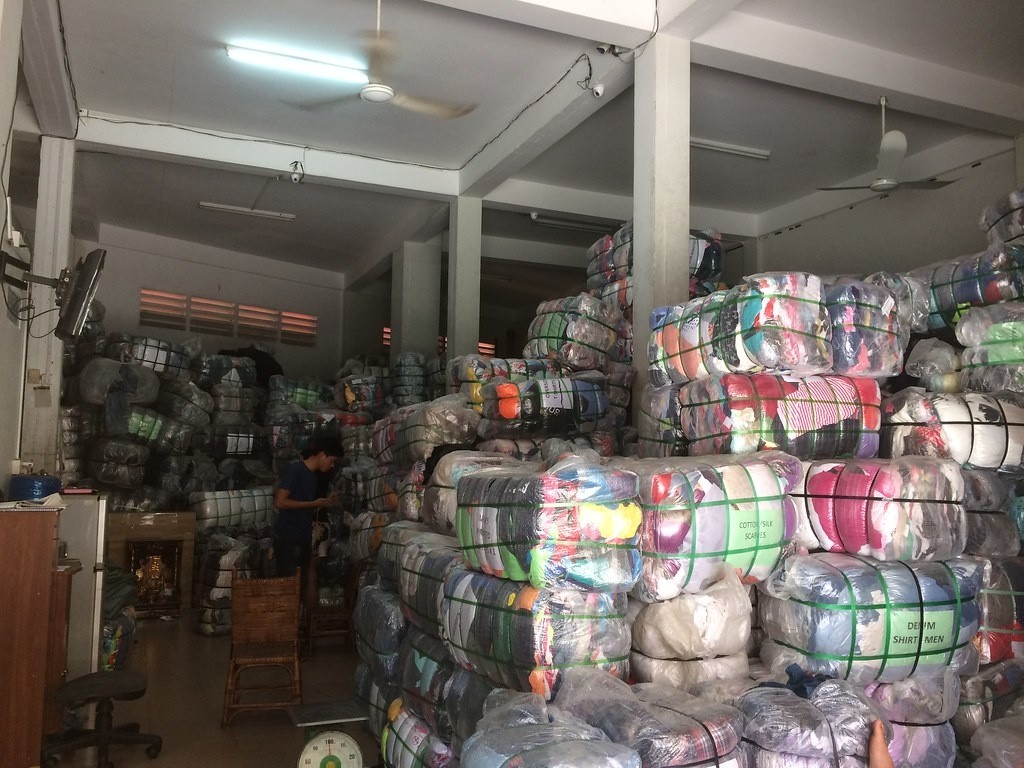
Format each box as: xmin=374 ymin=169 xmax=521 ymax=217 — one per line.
xmin=0 ymin=506 xmax=85 ymax=768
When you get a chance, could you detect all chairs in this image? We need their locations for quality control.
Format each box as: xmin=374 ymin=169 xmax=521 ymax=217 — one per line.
xmin=306 ymin=553 xmax=355 ymax=657
xmin=41 ymin=670 xmax=163 ymax=768
xmin=221 ymin=566 xmax=302 ymax=729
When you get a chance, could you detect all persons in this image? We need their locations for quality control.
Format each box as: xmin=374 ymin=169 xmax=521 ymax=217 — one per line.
xmin=274 ymin=436 xmax=344 ymax=609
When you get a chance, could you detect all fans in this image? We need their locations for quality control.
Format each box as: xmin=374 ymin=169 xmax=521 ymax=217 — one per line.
xmin=284 ymin=31 xmax=477 ymax=119
xmin=813 ymin=129 xmax=961 ymax=193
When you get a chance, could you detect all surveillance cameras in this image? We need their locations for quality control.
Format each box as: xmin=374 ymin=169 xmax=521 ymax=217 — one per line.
xmin=292 ymin=174 xmax=300 ymax=183
xmin=597 ymin=43 xmax=612 ymax=54
xmin=593 ymin=86 xmax=604 ymax=97
xmin=531 ymin=213 xmax=538 ymax=220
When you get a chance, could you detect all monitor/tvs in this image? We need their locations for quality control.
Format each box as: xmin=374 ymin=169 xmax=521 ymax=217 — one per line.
xmin=54 ymin=248 xmax=108 ymax=343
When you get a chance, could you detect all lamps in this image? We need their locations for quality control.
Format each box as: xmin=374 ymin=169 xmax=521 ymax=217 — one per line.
xmin=690 ymin=137 xmax=769 ymax=159
xmin=536 ymin=219 xmax=611 ymax=234
xmin=198 ymin=202 xmax=295 ymax=222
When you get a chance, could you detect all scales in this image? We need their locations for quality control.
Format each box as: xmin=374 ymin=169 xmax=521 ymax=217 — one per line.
xmin=284 ymin=699 xmax=371 ymax=768
